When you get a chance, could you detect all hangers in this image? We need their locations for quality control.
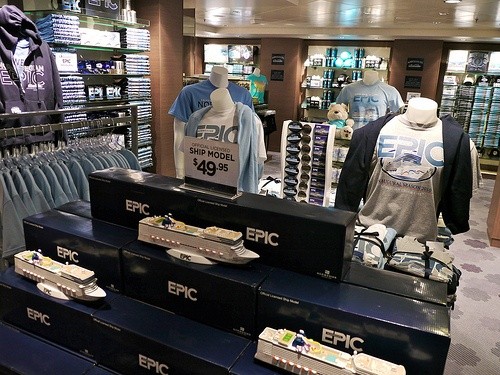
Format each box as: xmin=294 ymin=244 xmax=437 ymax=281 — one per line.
xmin=0 ymin=118 xmax=126 ymax=169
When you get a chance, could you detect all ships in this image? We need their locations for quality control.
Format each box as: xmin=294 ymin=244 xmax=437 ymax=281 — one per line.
xmin=14 ymin=250 xmax=107 ymax=305
xmin=255 ymin=325 xmax=406 ymax=375
xmin=138 ymin=213 xmax=260 ymax=267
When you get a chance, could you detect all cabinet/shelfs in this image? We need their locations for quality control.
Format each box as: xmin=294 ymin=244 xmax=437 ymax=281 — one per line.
xmin=24 ymin=10 xmax=155 ymax=170
xmin=301 ymin=40 xmax=393 ymax=123
xmin=435 ymin=42 xmax=500 ymax=157
